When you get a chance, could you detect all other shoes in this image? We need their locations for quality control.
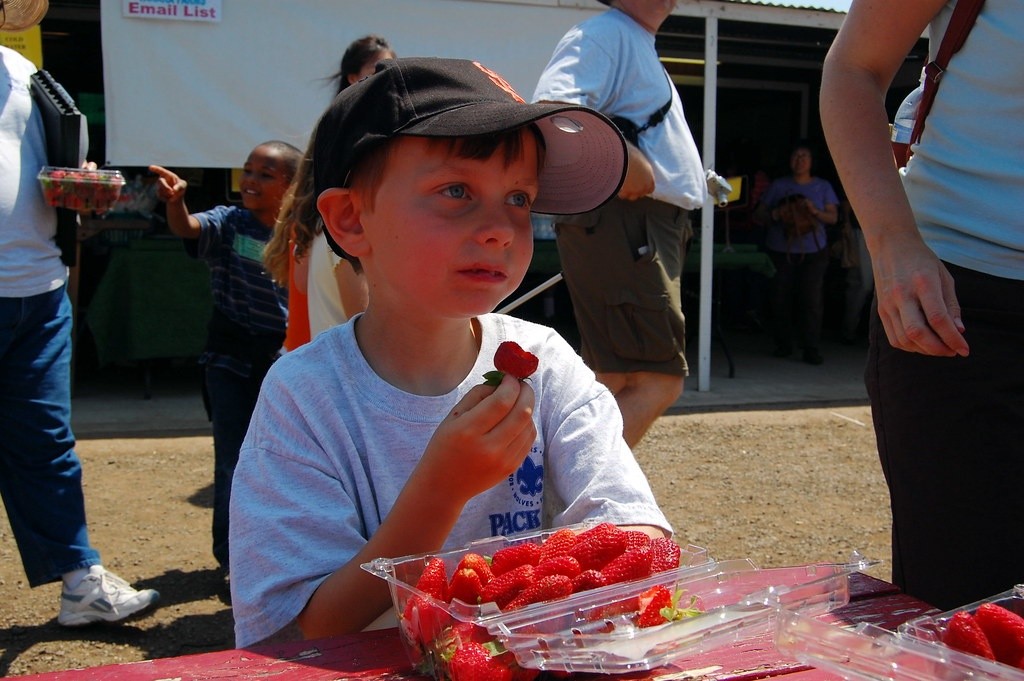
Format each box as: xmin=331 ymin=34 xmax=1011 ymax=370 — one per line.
xmin=216 ymin=561 xmax=231 ymax=593
xmin=773 ymin=346 xmax=794 ymax=360
xmin=802 ymin=351 xmax=821 ymax=366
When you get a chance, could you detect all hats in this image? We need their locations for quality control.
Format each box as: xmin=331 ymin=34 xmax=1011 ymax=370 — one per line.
xmin=313 ymin=58 xmax=628 ymax=216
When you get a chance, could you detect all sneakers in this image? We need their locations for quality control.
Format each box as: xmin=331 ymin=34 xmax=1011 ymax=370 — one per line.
xmin=57 ymin=564 xmax=159 ymax=627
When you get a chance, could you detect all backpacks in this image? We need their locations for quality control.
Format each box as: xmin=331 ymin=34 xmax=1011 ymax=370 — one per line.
xmin=779 ymin=189 xmax=828 ymax=265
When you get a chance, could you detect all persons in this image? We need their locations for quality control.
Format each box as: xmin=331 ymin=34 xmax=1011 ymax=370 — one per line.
xmin=0 ymin=0 xmax=160 ymax=625
xmin=319 ymin=35 xmax=397 ymax=98
xmin=751 ymin=0 xmax=1024 ymax=613
xmin=229 ymin=57 xmax=674 ymax=650
xmin=150 ymin=141 xmax=304 ymax=594
xmin=262 ymin=117 xmax=368 ymax=365
xmin=530 ymin=0 xmax=708 ymax=449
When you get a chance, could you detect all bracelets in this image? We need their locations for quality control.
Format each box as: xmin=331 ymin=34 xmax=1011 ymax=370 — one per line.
xmin=814 ymin=208 xmax=821 ymax=217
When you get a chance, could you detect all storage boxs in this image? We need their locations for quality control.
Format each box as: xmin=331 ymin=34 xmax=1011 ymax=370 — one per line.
xmin=360 ymin=517 xmax=1024 ymax=681
xmin=37 ymin=164 xmax=126 ymax=216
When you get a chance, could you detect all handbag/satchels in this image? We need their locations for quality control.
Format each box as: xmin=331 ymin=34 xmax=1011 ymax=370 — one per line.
xmin=607 ymin=116 xmax=639 ymax=150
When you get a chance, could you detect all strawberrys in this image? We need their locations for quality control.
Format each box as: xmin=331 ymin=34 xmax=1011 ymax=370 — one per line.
xmin=930 ymin=602 xmax=1024 ymax=681
xmin=44 ymin=169 xmax=123 ymax=214
xmin=401 ymin=521 xmax=680 ymax=681
xmin=494 ymin=340 xmax=539 ymax=381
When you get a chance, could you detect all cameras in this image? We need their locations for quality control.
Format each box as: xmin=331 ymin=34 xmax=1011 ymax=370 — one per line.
xmin=705 ymin=175 xmax=734 ymax=208
